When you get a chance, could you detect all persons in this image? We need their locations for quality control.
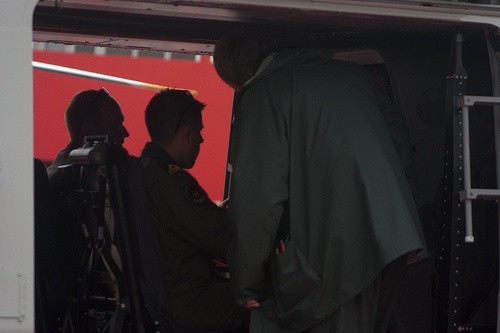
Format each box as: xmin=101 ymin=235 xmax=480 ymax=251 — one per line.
xmin=209 ymin=27 xmax=429 ymax=333
xmin=137 ymin=87 xmax=250 ymax=333
xmin=45 ymin=89 xmax=141 ymax=248
